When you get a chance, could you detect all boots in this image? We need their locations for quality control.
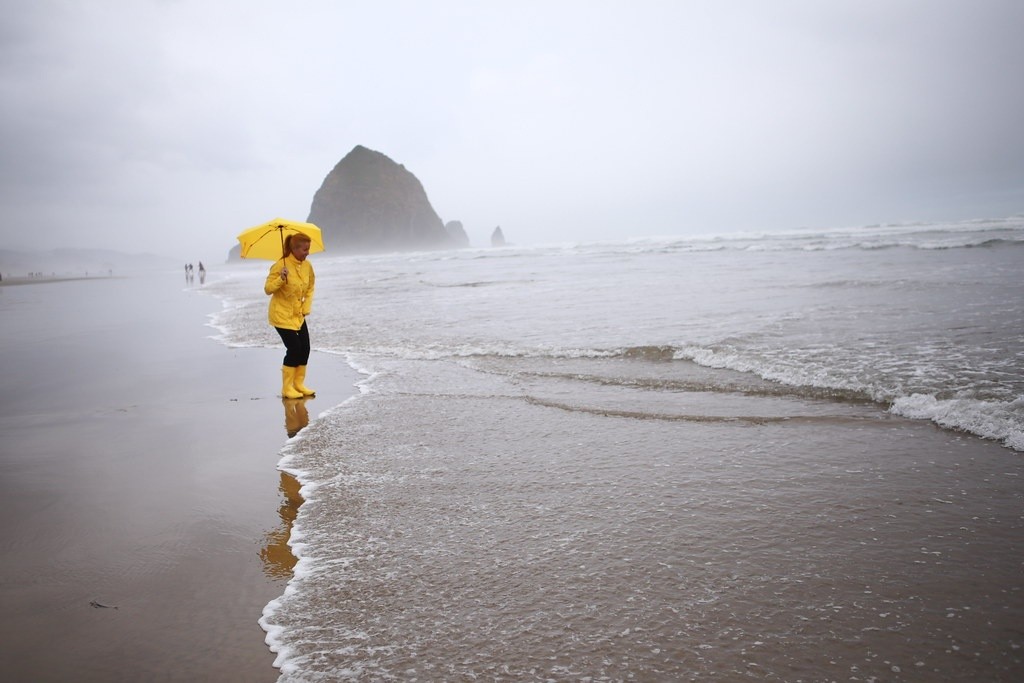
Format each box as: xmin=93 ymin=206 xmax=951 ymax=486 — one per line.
xmin=293 ymin=365 xmax=315 ymax=396
xmin=281 ymin=365 xmax=304 ymax=398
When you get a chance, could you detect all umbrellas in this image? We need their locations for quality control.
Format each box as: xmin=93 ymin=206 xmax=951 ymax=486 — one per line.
xmin=236 ymin=218 xmax=325 ymax=261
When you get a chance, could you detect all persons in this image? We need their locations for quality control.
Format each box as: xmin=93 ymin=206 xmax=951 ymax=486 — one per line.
xmin=184 ymin=260 xmax=207 ymax=274
xmin=264 ymin=232 xmax=317 ymax=401
